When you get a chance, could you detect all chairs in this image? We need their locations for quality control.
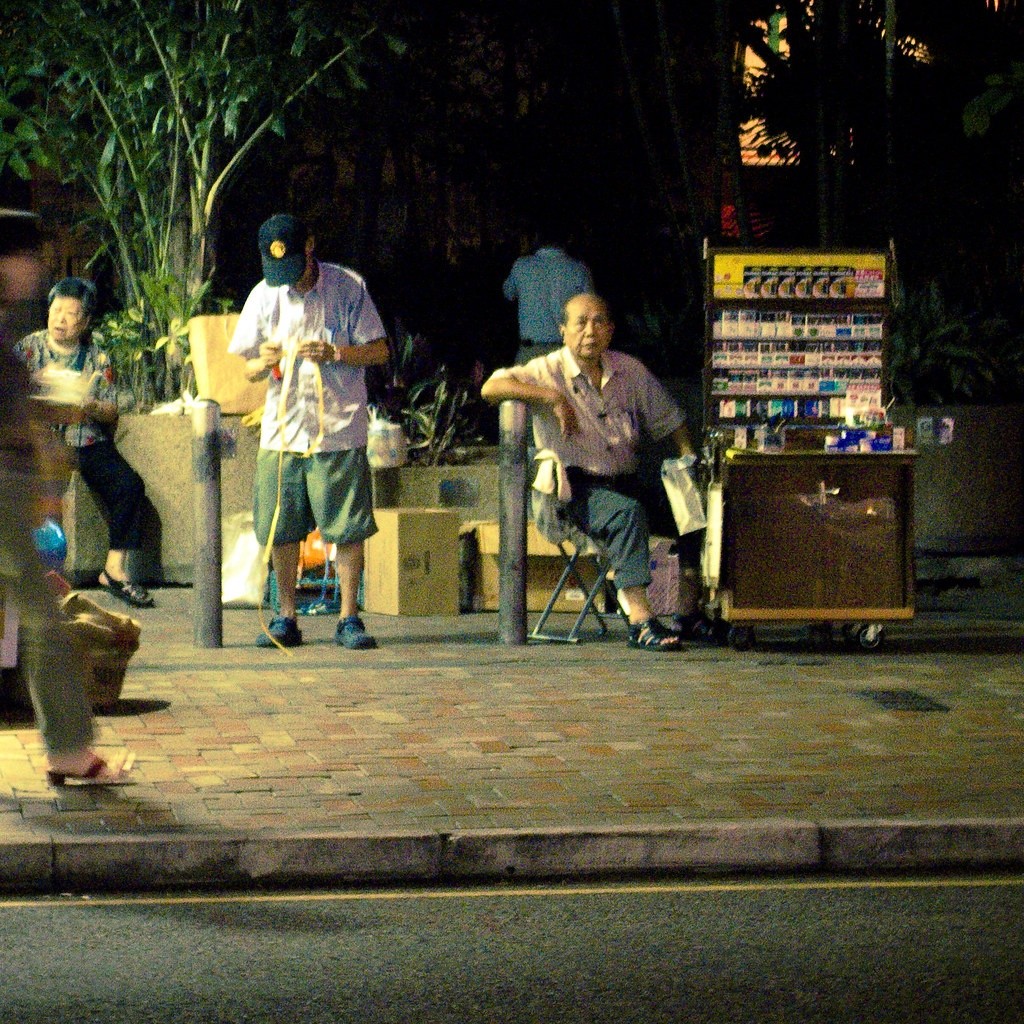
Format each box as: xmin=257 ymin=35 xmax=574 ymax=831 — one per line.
xmin=522 ymin=447 xmax=634 ymax=645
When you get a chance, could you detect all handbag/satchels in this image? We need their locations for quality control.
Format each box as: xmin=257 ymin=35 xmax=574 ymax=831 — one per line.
xmin=700 ymin=482 xmax=724 ymax=589
xmin=367 ymin=403 xmax=409 ymax=468
xmin=660 ymin=453 xmax=708 ymax=537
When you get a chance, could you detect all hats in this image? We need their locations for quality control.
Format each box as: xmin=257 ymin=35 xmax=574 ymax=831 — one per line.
xmin=258 ymin=214 xmax=305 ymax=287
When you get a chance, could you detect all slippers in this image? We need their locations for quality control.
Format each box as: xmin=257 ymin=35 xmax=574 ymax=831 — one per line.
xmin=45 ymin=757 xmax=133 ymax=787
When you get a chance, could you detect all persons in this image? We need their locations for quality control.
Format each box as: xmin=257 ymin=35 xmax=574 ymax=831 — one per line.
xmin=13 ymin=278 xmax=154 ymax=609
xmin=481 ymin=292 xmax=722 ymax=648
xmin=503 ymin=226 xmax=593 ymax=366
xmin=1 ymin=210 xmax=122 ymax=788
xmin=229 ymin=215 xmax=389 ymax=648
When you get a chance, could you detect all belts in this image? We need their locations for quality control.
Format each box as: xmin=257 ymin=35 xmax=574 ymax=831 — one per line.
xmin=520 ymin=339 xmax=563 ymax=348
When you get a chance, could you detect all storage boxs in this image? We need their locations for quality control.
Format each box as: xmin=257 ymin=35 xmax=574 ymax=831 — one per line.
xmin=459 ymin=516 xmax=605 ymax=616
xmin=646 ymin=534 xmax=684 ymax=620
xmin=361 ymin=507 xmax=464 ymax=618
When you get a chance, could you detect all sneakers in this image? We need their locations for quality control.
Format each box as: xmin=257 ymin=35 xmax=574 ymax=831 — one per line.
xmin=255 ymin=616 xmax=303 ymax=647
xmin=334 ymin=616 xmax=375 ymax=649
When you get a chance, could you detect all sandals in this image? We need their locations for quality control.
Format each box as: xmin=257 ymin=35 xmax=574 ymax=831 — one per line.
xmin=98 ymin=568 xmax=154 ymax=608
xmin=625 ymin=616 xmax=681 ymax=651
xmin=673 ymin=608 xmax=733 ymax=643
